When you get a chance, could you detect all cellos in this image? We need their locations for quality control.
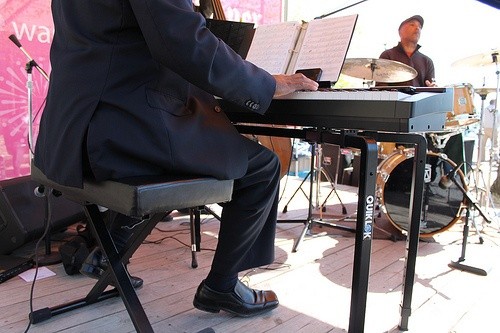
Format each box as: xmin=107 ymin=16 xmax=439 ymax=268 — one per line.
xmin=211 ymin=0 xmax=293 ymax=178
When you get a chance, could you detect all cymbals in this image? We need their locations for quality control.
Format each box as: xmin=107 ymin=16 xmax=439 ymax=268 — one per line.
xmin=341 ymin=58 xmax=418 ymax=83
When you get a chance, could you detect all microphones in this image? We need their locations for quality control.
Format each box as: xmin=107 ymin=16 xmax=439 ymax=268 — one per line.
xmin=439 ymin=162 xmax=465 ymax=189
xmin=34 ymin=183 xmax=46 ymax=197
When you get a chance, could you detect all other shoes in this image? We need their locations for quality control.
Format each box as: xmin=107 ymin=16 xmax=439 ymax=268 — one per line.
xmin=59 ymin=237 xmax=91 ymax=276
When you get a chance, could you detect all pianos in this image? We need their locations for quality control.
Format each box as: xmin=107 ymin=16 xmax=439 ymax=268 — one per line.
xmin=216 ymin=85 xmax=453 ymax=332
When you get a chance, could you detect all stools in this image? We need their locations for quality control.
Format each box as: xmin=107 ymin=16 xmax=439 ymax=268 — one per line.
xmin=28 ymin=160 xmax=235 ymax=333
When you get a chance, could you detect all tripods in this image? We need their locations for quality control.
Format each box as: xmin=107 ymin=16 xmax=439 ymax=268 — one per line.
xmin=277 ymin=143 xmax=356 ymax=253
xmin=465 ymin=96 xmax=500 ymax=232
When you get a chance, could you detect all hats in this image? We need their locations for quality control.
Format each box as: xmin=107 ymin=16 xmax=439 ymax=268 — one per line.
xmin=397 ymin=15 xmax=424 ymax=30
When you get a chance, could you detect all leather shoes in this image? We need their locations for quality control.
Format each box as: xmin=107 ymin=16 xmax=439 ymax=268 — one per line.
xmin=79 ymin=247 xmax=144 ymax=288
xmin=193 ymin=280 xmax=279 ymax=317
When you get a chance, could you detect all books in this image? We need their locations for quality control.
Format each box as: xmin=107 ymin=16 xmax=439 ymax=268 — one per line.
xmin=242 ymin=14 xmax=359 ymax=84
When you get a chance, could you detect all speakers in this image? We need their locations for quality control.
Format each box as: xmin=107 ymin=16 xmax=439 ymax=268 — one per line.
xmin=0 ymin=175 xmax=87 ymax=254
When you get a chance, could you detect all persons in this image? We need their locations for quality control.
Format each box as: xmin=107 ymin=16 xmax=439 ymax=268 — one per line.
xmin=34 ymin=0 xmax=319 ymax=317
xmin=376 ymin=15 xmax=455 ymax=175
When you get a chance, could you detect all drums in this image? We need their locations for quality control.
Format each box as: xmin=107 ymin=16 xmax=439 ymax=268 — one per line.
xmin=351 ymin=138 xmax=395 ymax=160
xmin=445 ymin=83 xmax=481 ymax=127
xmin=376 ymin=147 xmax=467 ymax=239
